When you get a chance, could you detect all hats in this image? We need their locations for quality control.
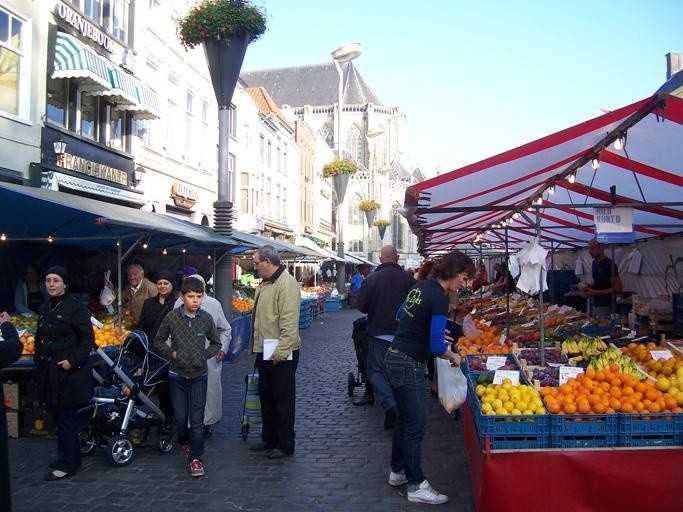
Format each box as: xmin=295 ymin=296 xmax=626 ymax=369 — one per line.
xmin=43 ymin=265 xmax=68 ymax=285
xmin=157 ymin=270 xmax=176 ymax=283
xmin=176 ymin=266 xmax=198 ymax=275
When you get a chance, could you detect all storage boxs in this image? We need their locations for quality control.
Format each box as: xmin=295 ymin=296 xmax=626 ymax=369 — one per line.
xmin=5 ymin=411 xmax=20 ymax=439
xmin=2 ymin=382 xmax=20 ymax=411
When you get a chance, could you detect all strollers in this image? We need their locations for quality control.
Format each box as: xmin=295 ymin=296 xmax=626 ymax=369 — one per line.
xmin=347 ymin=315 xmax=371 ymax=397
xmin=74 ymin=331 xmax=173 ymax=468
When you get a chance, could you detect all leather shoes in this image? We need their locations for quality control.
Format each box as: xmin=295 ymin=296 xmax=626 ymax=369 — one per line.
xmin=43 ymin=467 xmax=77 ymax=481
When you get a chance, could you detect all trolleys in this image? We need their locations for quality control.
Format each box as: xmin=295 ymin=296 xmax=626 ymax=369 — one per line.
xmin=238 ymin=356 xmax=265 ymax=443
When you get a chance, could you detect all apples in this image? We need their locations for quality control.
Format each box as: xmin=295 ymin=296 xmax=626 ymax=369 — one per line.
xmin=620 ymin=342 xmax=683 ymax=380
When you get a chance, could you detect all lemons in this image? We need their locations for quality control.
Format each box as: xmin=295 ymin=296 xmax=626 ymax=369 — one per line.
xmin=476 ymin=377 xmax=546 ymax=422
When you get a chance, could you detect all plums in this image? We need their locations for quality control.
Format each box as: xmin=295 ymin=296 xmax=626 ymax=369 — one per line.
xmin=470 ymin=359 xmax=517 ymax=370
xmin=518 ymin=348 xmax=568 ymax=386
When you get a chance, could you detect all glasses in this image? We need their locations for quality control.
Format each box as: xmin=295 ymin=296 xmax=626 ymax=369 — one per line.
xmin=255 ymin=261 xmax=264 ymax=265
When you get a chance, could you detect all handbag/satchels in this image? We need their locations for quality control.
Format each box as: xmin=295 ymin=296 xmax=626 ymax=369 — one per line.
xmin=60 ymin=364 xmax=95 ymax=409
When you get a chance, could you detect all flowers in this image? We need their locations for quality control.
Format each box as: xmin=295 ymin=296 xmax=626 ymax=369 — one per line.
xmin=174 ymin=0 xmax=267 ymax=50
xmin=357 ymin=199 xmax=381 ymax=217
xmin=369 ymin=216 xmax=392 ymax=232
xmin=322 ymin=158 xmax=357 ymax=181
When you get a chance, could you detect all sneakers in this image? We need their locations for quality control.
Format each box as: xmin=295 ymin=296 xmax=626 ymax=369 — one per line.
xmin=190 ymin=459 xmax=206 ymax=477
xmin=249 ymin=441 xmax=272 ymax=450
xmin=267 ymin=448 xmax=295 ymax=459
xmin=182 ymin=442 xmax=192 ymax=468
xmin=383 ymin=405 xmax=402 ymax=430
xmin=388 ymin=469 xmax=408 ymax=486
xmin=406 ymin=480 xmax=449 ymax=504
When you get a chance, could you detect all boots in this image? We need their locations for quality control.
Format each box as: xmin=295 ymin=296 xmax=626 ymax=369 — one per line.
xmin=352 ymin=376 xmax=375 ymax=406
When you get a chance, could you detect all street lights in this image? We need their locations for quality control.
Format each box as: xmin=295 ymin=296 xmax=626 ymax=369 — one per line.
xmin=365 ymin=126 xmax=385 ymax=249
xmin=329 ymin=41 xmax=363 ymax=304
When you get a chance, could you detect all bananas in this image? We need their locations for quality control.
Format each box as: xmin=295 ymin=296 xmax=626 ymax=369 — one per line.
xmin=562 ymin=335 xmax=648 ymax=382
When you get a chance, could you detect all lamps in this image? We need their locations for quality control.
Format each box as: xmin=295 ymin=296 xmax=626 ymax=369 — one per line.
xmin=46 ymin=132 xmax=68 ymax=161
xmin=132 ymin=162 xmax=147 ymax=188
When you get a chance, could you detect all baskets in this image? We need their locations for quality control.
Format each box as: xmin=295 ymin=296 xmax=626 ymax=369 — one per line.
xmin=467 ymin=392 xmax=550 ymax=450
xmin=466 ymin=371 xmax=550 ymax=435
xmin=619 ymin=412 xmax=683 ymax=433
xmin=619 ymin=432 xmax=683 ymax=446
xmin=465 ymin=353 xmax=522 ymax=372
xmin=551 ymin=434 xmax=619 ymax=449
xmin=537 ymin=390 xmax=619 ymax=436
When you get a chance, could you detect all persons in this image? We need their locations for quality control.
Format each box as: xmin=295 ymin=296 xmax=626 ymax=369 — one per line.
xmin=33 ymin=268 xmax=95 ymax=479
xmin=350 ymin=269 xmax=364 ymax=309
xmin=1 ymin=312 xmax=24 ymax=512
xmin=357 ymin=246 xmax=417 ymax=430
xmin=153 ymin=277 xmax=222 ymax=475
xmin=382 ymin=249 xmax=477 ymax=504
xmin=419 ymin=261 xmax=457 ymax=399
xmin=406 ymin=267 xmax=421 ymax=281
xmin=474 ymin=263 xmax=515 ymax=295
xmin=248 ymin=244 xmax=301 ymax=459
xmin=104 ymin=265 xmax=232 ymax=437
xmin=577 ymin=240 xmax=625 ymax=320
xmin=351 ymin=379 xmax=373 ymax=405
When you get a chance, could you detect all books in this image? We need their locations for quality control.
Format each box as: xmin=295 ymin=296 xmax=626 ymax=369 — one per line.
xmin=263 ymin=339 xmax=293 ymax=362
